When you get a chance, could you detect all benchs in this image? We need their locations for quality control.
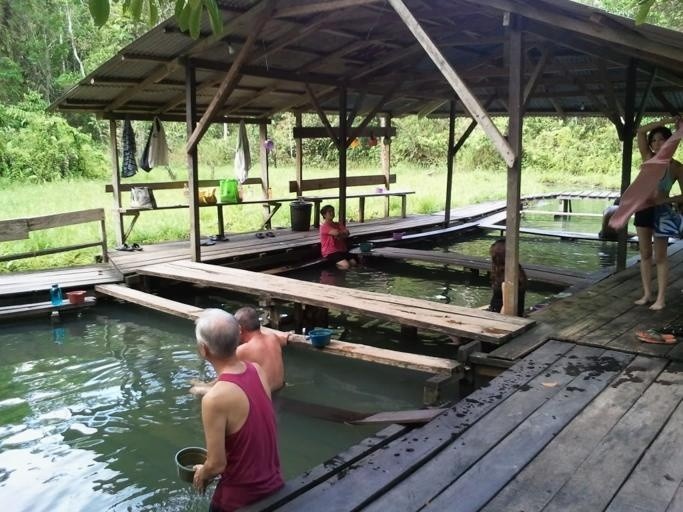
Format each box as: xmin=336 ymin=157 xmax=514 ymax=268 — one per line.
xmin=289 ymin=174 xmax=416 ymax=227
xmin=105 ymin=177 xmax=282 ymax=245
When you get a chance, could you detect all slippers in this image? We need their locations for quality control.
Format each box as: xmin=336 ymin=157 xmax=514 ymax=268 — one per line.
xmin=129 ymin=243 xmax=141 ymax=251
xmin=117 ymin=243 xmax=132 ymax=251
xmin=635 ymin=324 xmax=682 ymax=344
xmin=255 ymin=232 xmax=264 ymax=238
xmin=211 ymin=235 xmax=228 ymax=241
xmin=265 ymin=232 xmax=274 ymax=237
xmin=200 ymin=241 xmax=215 ymax=246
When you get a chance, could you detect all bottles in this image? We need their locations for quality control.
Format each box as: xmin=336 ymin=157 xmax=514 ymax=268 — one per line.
xmin=49 ymin=283 xmax=62 ymax=305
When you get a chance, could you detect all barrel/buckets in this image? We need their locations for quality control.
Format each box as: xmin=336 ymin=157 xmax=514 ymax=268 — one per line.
xmin=289 ymin=201 xmax=312 ymax=232
xmin=174 ymin=446 xmax=209 ymax=481
xmin=66 ymin=290 xmax=88 ymax=305
xmin=50 ymin=284 xmax=64 ymax=306
xmin=53 ymin=327 xmax=64 ymax=345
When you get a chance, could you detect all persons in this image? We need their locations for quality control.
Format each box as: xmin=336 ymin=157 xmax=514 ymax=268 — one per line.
xmin=319 ymin=205 xmax=357 ymax=269
xmin=632 ymin=115 xmax=682 ymax=310
xmin=598 ymin=197 xmax=637 ymax=240
xmin=475 ymin=237 xmax=527 ymax=315
xmin=188 ymin=306 xmax=283 ymax=512
xmin=187 ymin=307 xmax=288 ymax=394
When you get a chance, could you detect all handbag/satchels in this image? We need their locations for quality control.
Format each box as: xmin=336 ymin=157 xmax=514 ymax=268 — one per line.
xmin=218 ymin=178 xmax=239 ymax=204
xmin=197 ymin=188 xmax=216 ymax=205
xmin=139 ymin=120 xmax=160 ymax=172
xmin=130 ymin=186 xmax=156 ymax=209
xmin=147 ymin=116 xmax=169 ymax=168
